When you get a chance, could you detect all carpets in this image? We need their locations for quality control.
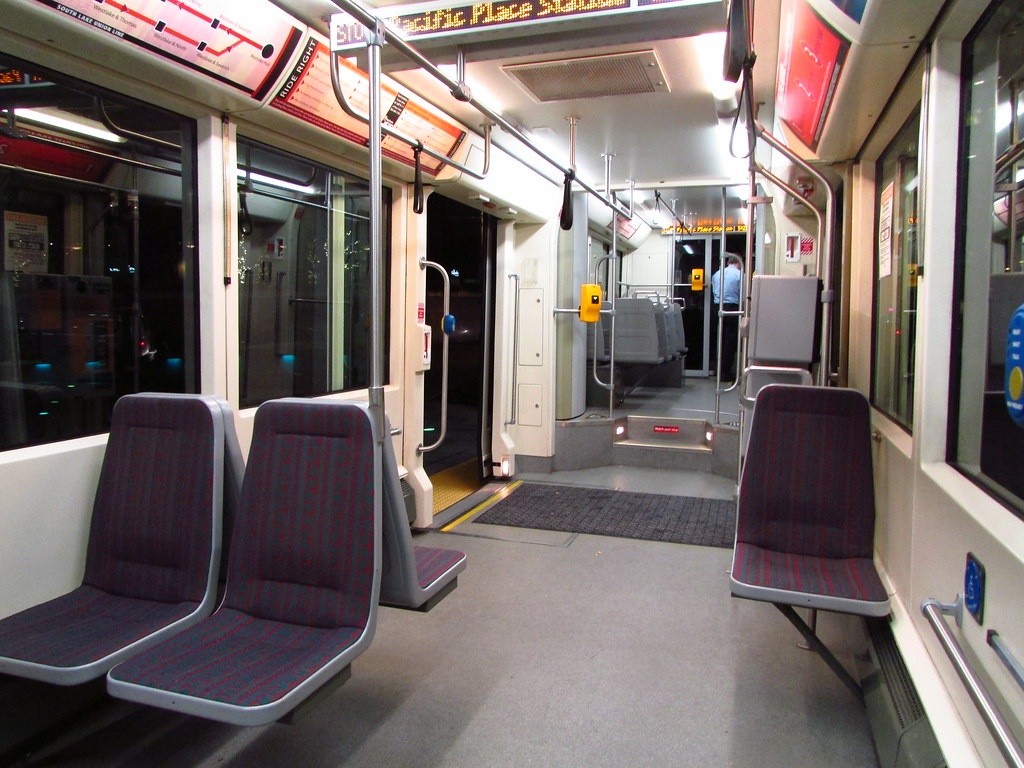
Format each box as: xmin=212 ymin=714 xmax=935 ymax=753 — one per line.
xmin=471 ymin=483 xmax=735 ymax=549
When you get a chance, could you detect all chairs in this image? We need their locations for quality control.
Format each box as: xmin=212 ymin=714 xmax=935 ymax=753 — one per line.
xmin=585 ymin=290 xmax=689 ymax=409
xmin=0 ymin=394 xmax=466 ymax=729
xmin=727 ymin=383 xmax=891 ymax=653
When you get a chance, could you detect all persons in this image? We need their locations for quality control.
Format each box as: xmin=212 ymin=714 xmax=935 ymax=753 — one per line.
xmin=712 ymin=251 xmax=745 ymax=381
